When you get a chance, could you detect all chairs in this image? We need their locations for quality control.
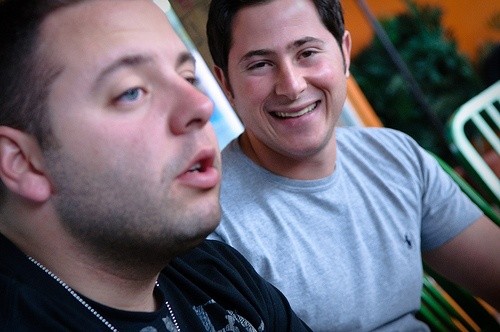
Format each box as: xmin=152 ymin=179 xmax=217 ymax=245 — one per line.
xmin=446 ymin=80 xmax=500 ymax=202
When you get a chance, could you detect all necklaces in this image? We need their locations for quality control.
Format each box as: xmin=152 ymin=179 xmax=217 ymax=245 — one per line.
xmin=26 ymin=254 xmax=181 ymax=332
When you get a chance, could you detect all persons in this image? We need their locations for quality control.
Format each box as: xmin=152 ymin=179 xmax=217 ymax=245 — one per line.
xmin=0 ymin=0 xmax=315 ymax=332
xmin=205 ymin=0 xmax=500 ymax=332
